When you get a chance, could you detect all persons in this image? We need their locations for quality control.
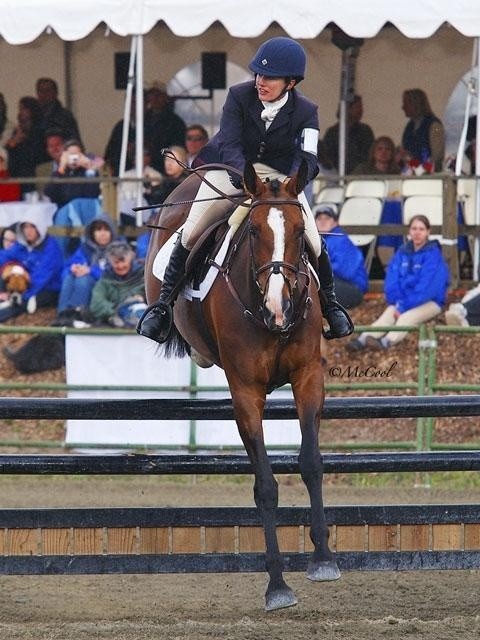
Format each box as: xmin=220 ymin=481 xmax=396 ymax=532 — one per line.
xmin=0 ymin=78 xmax=480 ymax=353
xmin=139 ymin=37 xmax=353 ymax=339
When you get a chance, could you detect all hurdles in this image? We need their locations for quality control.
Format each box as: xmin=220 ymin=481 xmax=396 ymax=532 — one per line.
xmin=0 ymin=395 xmax=480 ymax=575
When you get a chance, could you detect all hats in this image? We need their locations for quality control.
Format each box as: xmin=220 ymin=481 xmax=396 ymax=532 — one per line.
xmin=314 ymin=206 xmax=334 ymax=218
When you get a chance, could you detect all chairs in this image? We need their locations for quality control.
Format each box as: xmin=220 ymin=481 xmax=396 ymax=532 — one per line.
xmin=57 ymin=197 xmax=99 ymax=225
xmin=312 ymin=178 xmax=479 ymax=266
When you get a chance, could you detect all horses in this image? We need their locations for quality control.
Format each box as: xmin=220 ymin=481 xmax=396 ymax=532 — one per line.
xmin=143 ymin=165 xmax=342 ymax=612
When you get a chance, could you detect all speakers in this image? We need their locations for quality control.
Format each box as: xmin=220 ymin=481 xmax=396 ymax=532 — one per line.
xmin=201 ymin=51 xmax=227 ymax=89
xmin=114 ymin=51 xmax=137 ymax=88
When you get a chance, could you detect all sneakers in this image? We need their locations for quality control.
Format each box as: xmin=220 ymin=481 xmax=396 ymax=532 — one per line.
xmin=366 ymin=335 xmax=386 ymax=354
xmin=346 ymin=339 xmax=363 ymax=353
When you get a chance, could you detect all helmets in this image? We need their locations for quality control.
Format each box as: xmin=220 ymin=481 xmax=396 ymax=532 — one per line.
xmin=248 ymin=36 xmax=306 ymax=78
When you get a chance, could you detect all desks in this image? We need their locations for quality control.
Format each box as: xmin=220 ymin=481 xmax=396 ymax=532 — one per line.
xmin=2 ymin=199 xmax=56 ymax=230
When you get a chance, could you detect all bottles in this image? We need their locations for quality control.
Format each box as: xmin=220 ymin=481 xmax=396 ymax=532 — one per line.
xmin=83 ymin=152 xmax=99 ymax=178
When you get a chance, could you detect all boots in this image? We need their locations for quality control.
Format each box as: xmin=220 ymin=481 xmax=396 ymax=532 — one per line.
xmin=317 ymin=246 xmax=349 ymax=336
xmin=141 ymin=234 xmax=190 ymax=343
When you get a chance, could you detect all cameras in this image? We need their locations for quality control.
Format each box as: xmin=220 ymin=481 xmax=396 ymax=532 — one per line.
xmin=67 ymin=153 xmax=80 ymax=165
